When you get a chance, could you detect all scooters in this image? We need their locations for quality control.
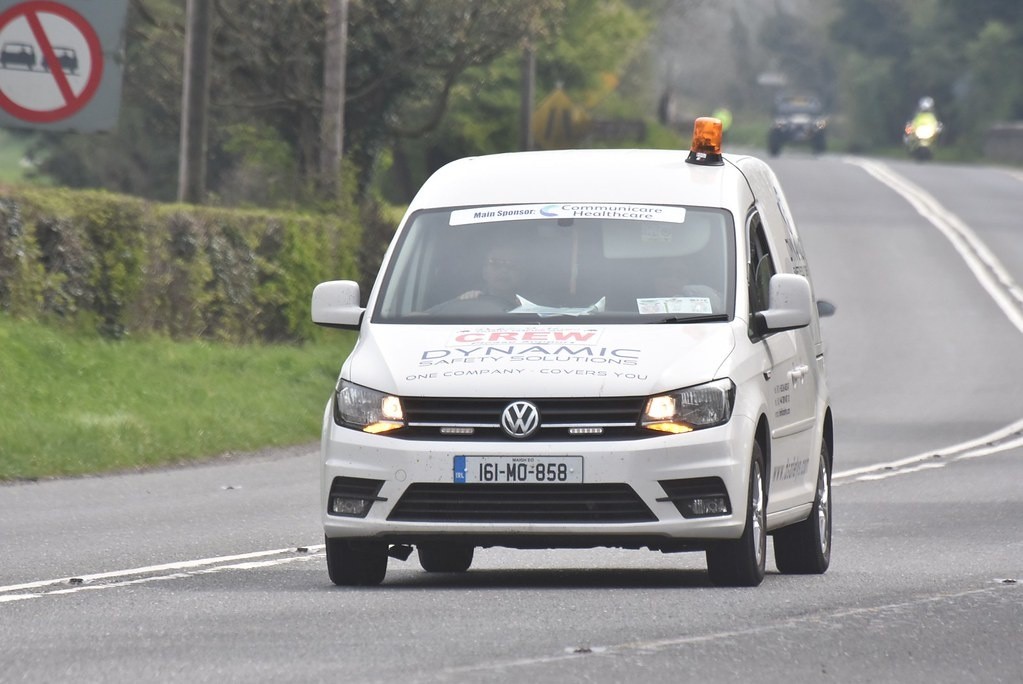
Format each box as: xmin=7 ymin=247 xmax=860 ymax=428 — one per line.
xmin=904 ymin=113 xmax=942 ymax=163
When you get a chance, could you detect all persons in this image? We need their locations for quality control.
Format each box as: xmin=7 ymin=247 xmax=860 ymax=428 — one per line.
xmin=905 ymin=97 xmax=942 ymax=135
xmin=460 ymin=246 xmax=528 ymax=304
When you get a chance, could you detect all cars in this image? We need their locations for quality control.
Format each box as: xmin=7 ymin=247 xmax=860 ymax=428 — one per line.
xmin=310 ymin=115 xmax=834 ymax=589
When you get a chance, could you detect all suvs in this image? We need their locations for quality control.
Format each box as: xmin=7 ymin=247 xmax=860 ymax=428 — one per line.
xmin=766 ymin=96 xmax=827 ymax=155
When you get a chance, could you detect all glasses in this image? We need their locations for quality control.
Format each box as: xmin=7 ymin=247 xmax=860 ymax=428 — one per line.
xmin=483 ymin=258 xmax=521 ymax=270
